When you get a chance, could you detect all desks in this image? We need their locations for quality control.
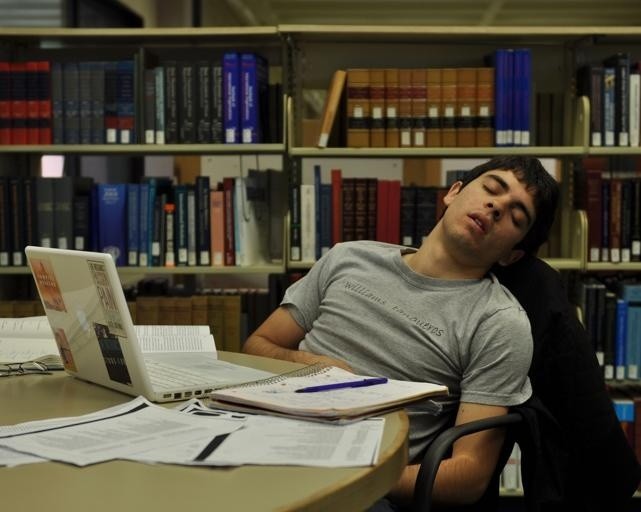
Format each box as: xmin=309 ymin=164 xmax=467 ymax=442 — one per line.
xmin=1 ymin=346 xmax=410 ymax=512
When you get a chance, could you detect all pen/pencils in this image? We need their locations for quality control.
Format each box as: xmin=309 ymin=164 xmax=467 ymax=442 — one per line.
xmin=294 ymin=378 xmax=388 ymax=393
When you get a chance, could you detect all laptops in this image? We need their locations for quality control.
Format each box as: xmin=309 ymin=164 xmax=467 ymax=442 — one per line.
xmin=24 ymin=246 xmax=279 ymax=403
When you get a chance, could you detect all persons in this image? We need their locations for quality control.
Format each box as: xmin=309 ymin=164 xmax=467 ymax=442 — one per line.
xmin=240 ymin=154 xmax=560 ymax=502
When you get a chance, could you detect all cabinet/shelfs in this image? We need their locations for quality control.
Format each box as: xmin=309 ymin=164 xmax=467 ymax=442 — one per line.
xmin=1 ymin=25 xmax=641 ymax=497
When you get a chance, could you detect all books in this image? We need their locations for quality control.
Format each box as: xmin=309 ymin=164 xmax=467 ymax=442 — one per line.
xmin=613 ymin=396 xmax=641 ymax=456
xmin=318 ymin=48 xmax=532 ymax=148
xmin=212 ymin=361 xmax=451 ymax=426
xmin=127 ymin=273 xmax=310 ymax=360
xmin=0 ymin=44 xmax=282 ymax=144
xmin=582 ymin=170 xmax=641 ymax=263
xmin=1 ymin=299 xmax=64 ymax=373
xmin=571 ymin=271 xmax=641 ymax=383
xmin=576 ymin=51 xmax=641 ymax=148
xmin=0 ymin=159 xmax=273 ymax=269
xmin=289 ymin=155 xmax=449 ymax=263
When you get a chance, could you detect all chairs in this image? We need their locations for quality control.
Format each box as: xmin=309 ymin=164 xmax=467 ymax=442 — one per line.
xmin=388 ymin=254 xmax=576 ymax=512
